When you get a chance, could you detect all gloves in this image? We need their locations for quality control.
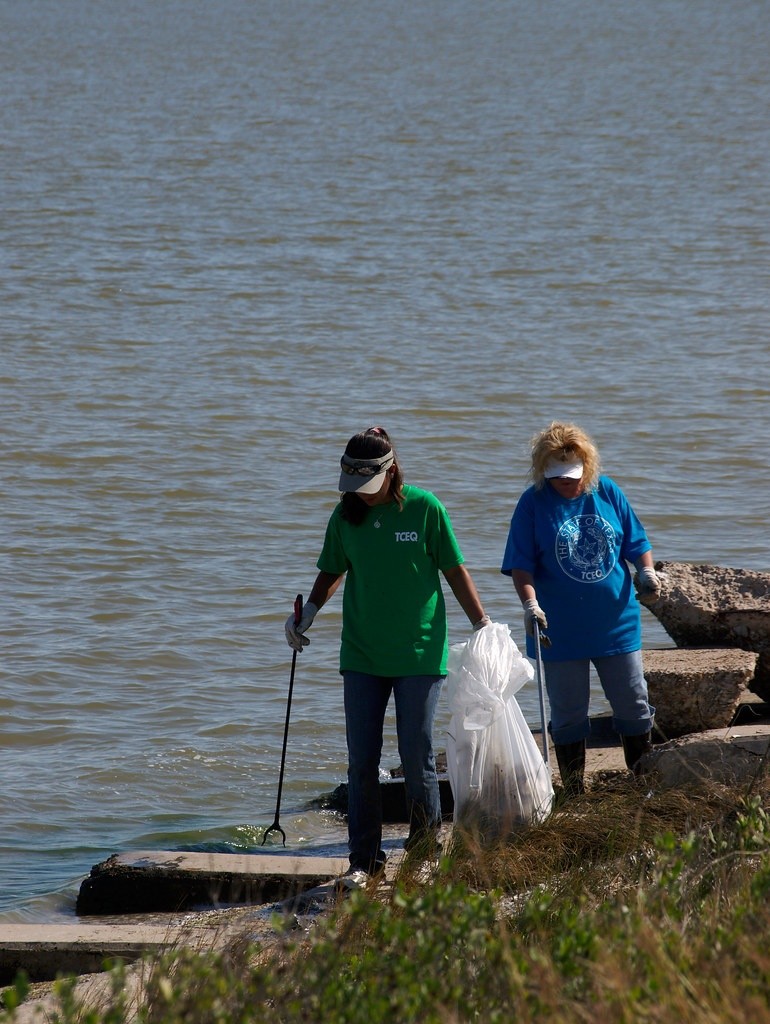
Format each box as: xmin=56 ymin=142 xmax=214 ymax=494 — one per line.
xmin=522 ymin=599 xmax=551 ymax=648
xmin=473 ymin=617 xmax=493 ymax=629
xmin=634 ymin=566 xmax=662 ymax=604
xmin=284 ymin=603 xmax=317 ymax=653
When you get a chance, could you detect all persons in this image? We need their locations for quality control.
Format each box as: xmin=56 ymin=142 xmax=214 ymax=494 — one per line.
xmin=285 ymin=428 xmax=494 ymax=892
xmin=501 ymin=419 xmax=662 ymax=806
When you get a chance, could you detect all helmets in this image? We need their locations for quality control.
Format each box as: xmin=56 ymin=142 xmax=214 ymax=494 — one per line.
xmin=338 ymin=447 xmax=393 ymax=494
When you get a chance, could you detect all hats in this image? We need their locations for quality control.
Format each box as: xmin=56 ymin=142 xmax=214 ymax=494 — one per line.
xmin=543 ymin=452 xmax=583 ymax=480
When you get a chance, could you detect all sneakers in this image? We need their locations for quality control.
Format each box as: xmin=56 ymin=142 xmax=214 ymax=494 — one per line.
xmin=403 ymin=859 xmax=439 ymax=885
xmin=333 ymin=866 xmax=385 ymax=891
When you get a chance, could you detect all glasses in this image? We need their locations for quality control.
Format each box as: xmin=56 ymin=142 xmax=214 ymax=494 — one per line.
xmin=340 ymin=455 xmax=394 ymax=476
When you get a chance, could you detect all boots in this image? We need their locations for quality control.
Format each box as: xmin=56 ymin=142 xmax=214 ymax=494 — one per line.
xmin=555 ymin=738 xmax=587 ymax=798
xmin=618 ymin=732 xmax=658 ymax=769
